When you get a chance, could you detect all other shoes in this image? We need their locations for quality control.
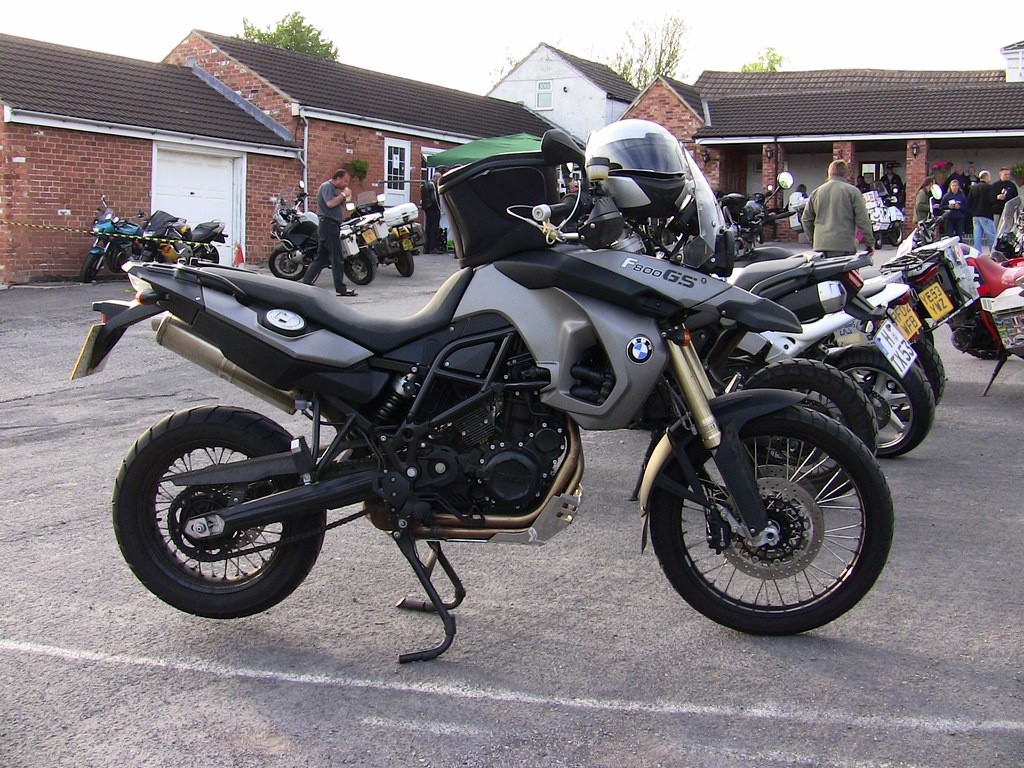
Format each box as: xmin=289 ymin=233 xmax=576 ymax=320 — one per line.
xmin=336 ymin=290 xmax=358 ymax=296
xmin=431 ymin=248 xmax=443 ymax=254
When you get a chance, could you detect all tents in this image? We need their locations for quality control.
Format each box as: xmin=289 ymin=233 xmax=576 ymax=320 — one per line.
xmin=427 ymin=132 xmax=544 ymax=168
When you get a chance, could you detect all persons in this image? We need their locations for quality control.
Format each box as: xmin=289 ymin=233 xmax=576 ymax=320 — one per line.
xmin=912 ymin=177 xmax=940 ymax=224
xmin=939 ymin=180 xmax=968 ymax=242
xmin=855 ymin=175 xmax=870 ymax=194
xmin=421 ymin=172 xmax=444 ymax=253
xmin=943 ymin=165 xmax=980 ymax=199
xmin=799 ymin=160 xmax=875 ymax=258
xmin=967 ymin=170 xmax=999 ymax=252
xmin=880 ymin=162 xmax=905 ymax=208
xmin=989 ymin=167 xmax=1019 ymax=226
xmin=302 ymin=169 xmax=359 ymax=296
xmin=784 ymin=183 xmax=808 ymax=234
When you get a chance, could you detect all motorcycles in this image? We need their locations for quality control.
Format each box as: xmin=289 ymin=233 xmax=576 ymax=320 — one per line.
xmin=861 ymin=181 xmax=905 ymax=250
xmin=897 ymin=184 xmax=1024 ymax=397
xmin=268 ymin=181 xmax=389 ymax=286
xmin=71 ymin=119 xmax=956 ymax=664
xmin=80 ymin=194 xmax=145 ymax=283
xmin=137 ymin=210 xmax=229 ymax=263
xmin=346 ymin=191 xmax=426 ymax=278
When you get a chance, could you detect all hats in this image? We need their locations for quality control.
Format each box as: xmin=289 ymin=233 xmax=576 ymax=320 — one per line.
xmin=887 ymin=164 xmax=893 ymax=169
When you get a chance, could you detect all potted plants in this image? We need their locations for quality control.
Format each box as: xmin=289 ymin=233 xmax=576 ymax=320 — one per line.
xmin=343 ymin=157 xmax=369 ymax=180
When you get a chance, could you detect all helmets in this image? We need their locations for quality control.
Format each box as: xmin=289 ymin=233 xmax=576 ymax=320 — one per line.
xmin=584 ymin=119 xmax=687 ymax=221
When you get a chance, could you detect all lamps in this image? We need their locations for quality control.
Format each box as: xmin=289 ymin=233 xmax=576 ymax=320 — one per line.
xmin=912 ymin=141 xmax=920 ymax=159
xmin=764 ymin=144 xmax=776 ymax=160
xmin=701 ymin=150 xmax=711 ymax=165
